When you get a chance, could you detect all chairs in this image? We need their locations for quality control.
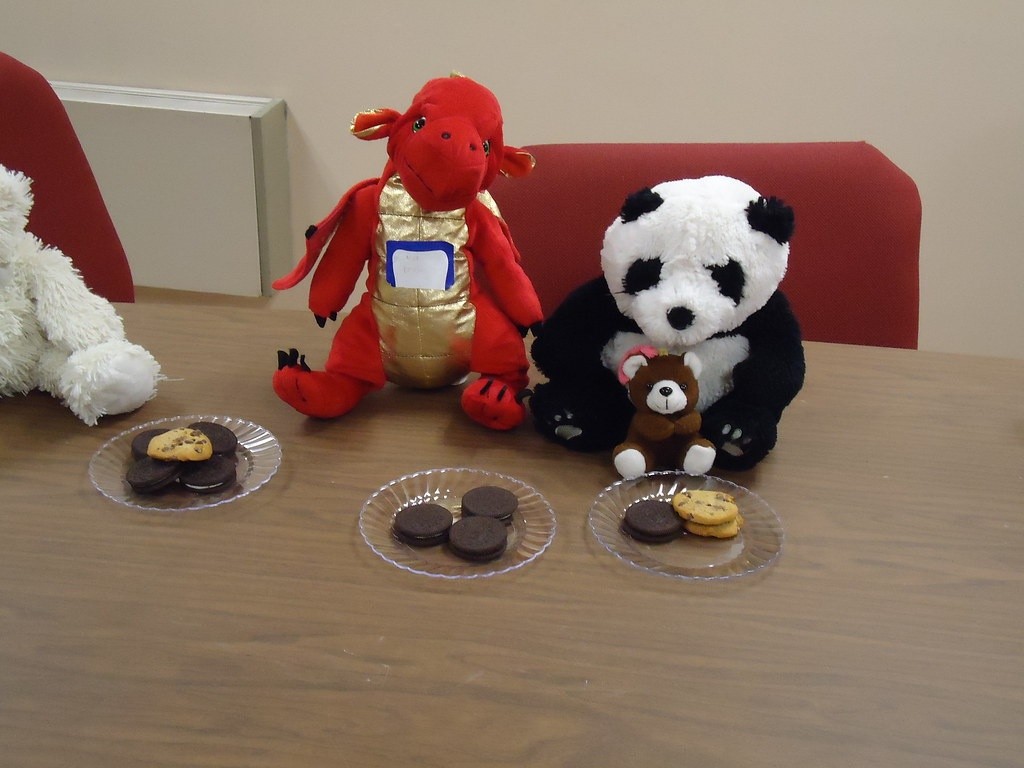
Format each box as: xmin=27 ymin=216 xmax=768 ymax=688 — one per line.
xmin=492 ymin=141 xmax=922 ymax=350
xmin=0 ymin=51 xmax=136 ymax=303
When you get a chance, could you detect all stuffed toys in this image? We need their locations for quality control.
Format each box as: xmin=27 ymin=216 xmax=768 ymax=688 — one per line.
xmin=0 ymin=164 xmax=163 ymax=427
xmin=612 ymin=349 xmax=717 ymax=481
xmin=269 ymin=76 xmax=541 ymax=431
xmin=530 ymin=176 xmax=806 ymax=472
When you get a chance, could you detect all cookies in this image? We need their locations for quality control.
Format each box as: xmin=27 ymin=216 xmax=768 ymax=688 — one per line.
xmin=623 ymin=490 xmax=744 ymax=544
xmin=126 ymin=421 xmax=238 ymax=494
xmin=393 ymin=486 xmax=519 ymax=561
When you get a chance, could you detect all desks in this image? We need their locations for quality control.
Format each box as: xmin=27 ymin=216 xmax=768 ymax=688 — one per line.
xmin=1 ymin=301 xmax=1024 ymax=768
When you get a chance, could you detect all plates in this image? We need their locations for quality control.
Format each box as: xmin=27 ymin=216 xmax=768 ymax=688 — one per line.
xmin=357 ymin=466 xmax=557 ymax=580
xmin=586 ymin=471 xmax=786 ymax=583
xmin=87 ymin=413 xmax=283 ymax=512
